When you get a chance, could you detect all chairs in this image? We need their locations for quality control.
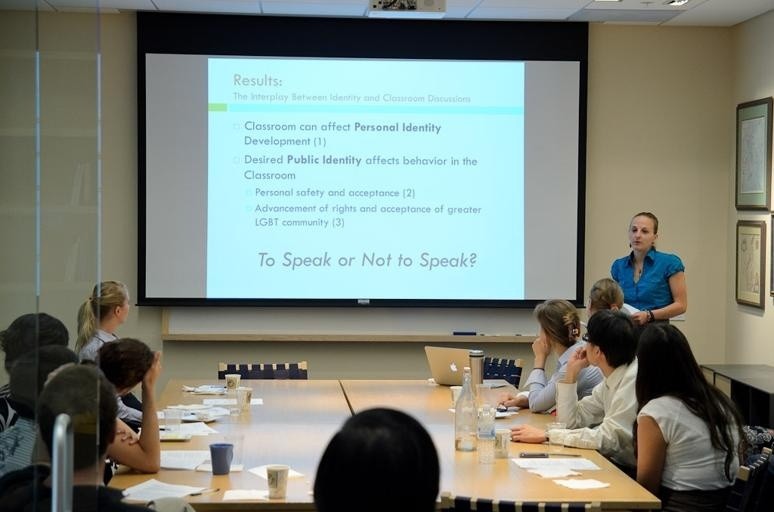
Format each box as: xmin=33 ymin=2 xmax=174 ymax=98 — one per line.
xmin=479 ymin=355 xmax=526 ymax=389
xmin=216 ymin=358 xmax=309 ymax=382
xmin=727 ymin=445 xmax=774 ymax=512
xmin=435 ymin=486 xmax=610 ymax=512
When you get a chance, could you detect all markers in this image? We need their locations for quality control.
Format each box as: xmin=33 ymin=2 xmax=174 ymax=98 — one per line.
xmin=512 ymin=334 xmax=535 ymax=337
xmin=480 ymin=333 xmax=502 ymax=337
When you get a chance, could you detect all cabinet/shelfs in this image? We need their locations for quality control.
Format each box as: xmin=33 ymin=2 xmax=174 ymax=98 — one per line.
xmin=697 ymin=354 xmax=773 ymax=430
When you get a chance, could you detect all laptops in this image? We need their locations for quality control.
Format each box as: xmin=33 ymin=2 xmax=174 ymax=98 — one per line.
xmin=424 ymin=345 xmax=503 ymax=388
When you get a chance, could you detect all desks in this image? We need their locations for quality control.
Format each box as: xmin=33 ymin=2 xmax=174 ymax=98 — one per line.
xmin=102 ymin=374 xmax=354 ymax=512
xmin=339 ymin=378 xmax=664 ymax=512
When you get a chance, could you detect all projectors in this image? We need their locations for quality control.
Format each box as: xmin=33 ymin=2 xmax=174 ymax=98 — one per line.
xmin=369 ymin=0 xmax=446 ymax=18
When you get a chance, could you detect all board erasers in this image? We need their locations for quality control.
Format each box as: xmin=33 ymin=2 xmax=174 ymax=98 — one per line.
xmin=452 ymin=331 xmax=478 ymax=337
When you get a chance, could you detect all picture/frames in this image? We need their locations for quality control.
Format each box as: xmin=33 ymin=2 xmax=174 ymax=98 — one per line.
xmin=731 ymin=94 xmax=773 ymax=213
xmin=731 ymin=217 xmax=769 ymax=313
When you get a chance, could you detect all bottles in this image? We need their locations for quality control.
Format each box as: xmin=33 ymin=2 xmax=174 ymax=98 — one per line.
xmin=454 ymin=367 xmax=478 ymax=450
xmin=468 ymin=349 xmax=489 ymax=385
xmin=495 ymin=428 xmax=509 ymax=458
xmin=477 ymin=404 xmax=495 ymax=465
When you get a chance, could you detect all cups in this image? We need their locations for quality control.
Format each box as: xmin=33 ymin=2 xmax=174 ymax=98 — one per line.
xmin=225 ymin=373 xmax=253 ymax=414
xmin=546 ymin=422 xmax=566 ymax=452
xmin=163 ymin=408 xmax=184 ymax=437
xmin=473 ymin=383 xmax=491 ymax=405
xmin=267 ymin=464 xmax=289 ymax=497
xmin=449 ymin=384 xmax=467 ymax=408
xmin=209 ymin=443 xmax=232 ymax=474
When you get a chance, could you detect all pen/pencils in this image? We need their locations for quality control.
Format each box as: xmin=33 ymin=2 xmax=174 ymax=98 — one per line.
xmin=210 ymin=385 xmax=227 ymax=389
xmin=159 ymin=427 xmax=171 ymax=432
xmin=185 ymin=413 xmax=198 ymax=416
xmin=187 ymin=488 xmax=220 ymax=497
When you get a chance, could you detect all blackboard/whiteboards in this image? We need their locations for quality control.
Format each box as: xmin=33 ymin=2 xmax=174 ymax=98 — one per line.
xmin=160 ymin=308 xmax=603 ymax=343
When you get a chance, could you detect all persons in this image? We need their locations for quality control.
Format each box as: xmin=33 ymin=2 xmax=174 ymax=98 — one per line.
xmin=313 ymin=408 xmax=440 ymax=511
xmin=498 ymin=279 xmax=742 ymax=511
xmin=610 ymin=212 xmax=687 ymax=327
xmin=0 ymin=281 xmax=161 ymax=512
